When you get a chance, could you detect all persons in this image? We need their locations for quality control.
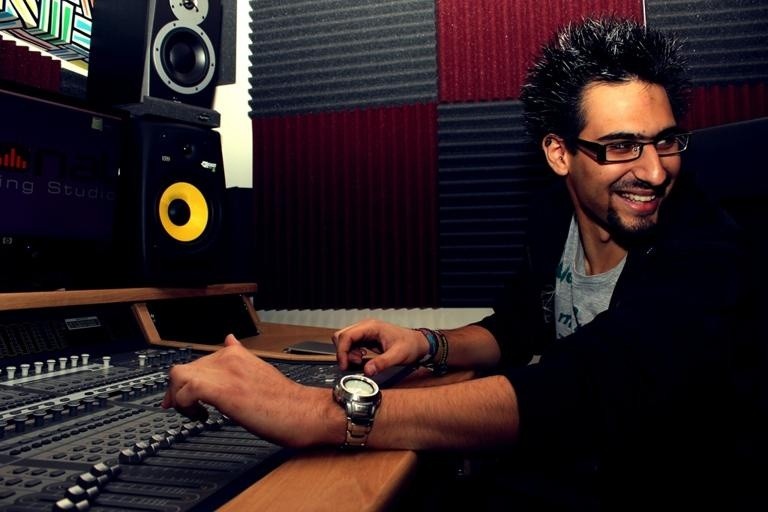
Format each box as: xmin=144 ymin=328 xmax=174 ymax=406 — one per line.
xmin=151 ymin=13 xmax=766 ymax=512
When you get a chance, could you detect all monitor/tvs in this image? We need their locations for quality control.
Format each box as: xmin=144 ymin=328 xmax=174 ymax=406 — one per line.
xmin=0 ymin=89 xmax=126 ymax=246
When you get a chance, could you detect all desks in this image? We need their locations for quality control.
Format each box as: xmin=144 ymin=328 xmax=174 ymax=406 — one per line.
xmin=2 ymin=280 xmax=472 ymax=511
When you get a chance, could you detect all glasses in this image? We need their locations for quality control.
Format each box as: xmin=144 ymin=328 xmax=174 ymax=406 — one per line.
xmin=574 ymin=129 xmax=690 ymax=165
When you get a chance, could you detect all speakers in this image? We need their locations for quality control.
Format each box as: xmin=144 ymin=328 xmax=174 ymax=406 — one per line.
xmin=121 ymin=121 xmax=227 ymax=288
xmin=88 ymin=0 xmax=223 ymax=119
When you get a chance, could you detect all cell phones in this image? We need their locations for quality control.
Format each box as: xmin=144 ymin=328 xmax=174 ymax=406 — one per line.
xmin=288 ymin=341 xmax=336 ymax=355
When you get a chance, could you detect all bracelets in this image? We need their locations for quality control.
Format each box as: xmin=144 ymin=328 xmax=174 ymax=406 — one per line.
xmin=415 ymin=326 xmax=450 ymax=374
xmin=332 ymin=374 xmax=382 ymax=454
